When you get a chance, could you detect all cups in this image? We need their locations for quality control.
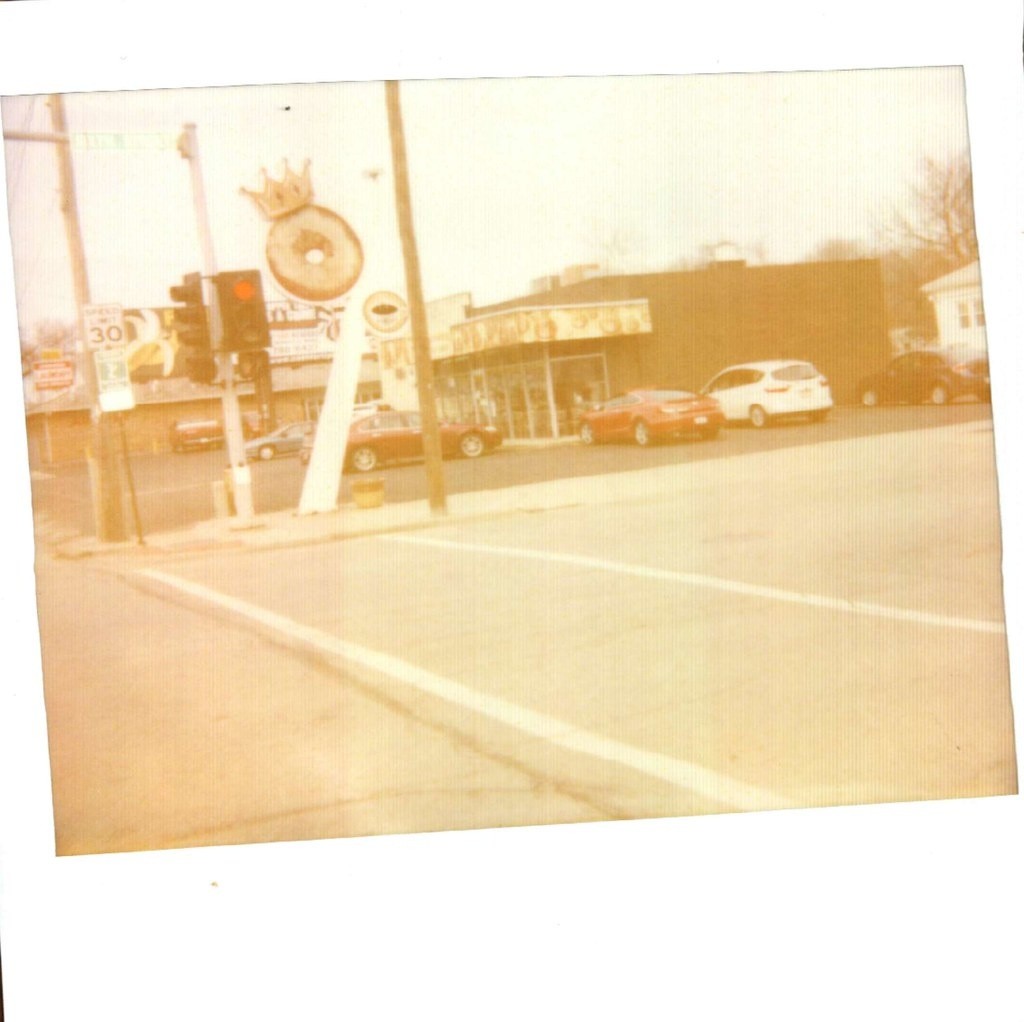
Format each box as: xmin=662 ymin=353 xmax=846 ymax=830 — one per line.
xmin=370 ymin=304 xmax=407 ymax=328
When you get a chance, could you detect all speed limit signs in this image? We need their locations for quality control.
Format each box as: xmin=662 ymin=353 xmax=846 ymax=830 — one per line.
xmin=81 ymin=304 xmax=126 ymax=348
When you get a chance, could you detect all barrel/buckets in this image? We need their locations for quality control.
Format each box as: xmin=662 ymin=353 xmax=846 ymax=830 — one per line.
xmin=349 ymin=476 xmax=387 ymax=508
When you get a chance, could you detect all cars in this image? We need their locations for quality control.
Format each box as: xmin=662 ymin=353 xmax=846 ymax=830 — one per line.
xmin=243 ymin=421 xmax=336 ymax=460
xmin=342 ymin=409 xmax=502 ymax=471
xmin=168 ymin=416 xmax=221 ymax=451
xmin=698 ymin=359 xmax=834 ymax=430
xmin=855 ymin=340 xmax=990 ymax=407
xmin=578 ymin=389 xmax=724 ymax=447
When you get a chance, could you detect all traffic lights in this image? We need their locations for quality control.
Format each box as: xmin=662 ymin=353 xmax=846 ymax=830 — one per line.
xmin=168 ymin=272 xmax=210 ymax=351
xmin=217 ymin=269 xmax=271 ymax=352
